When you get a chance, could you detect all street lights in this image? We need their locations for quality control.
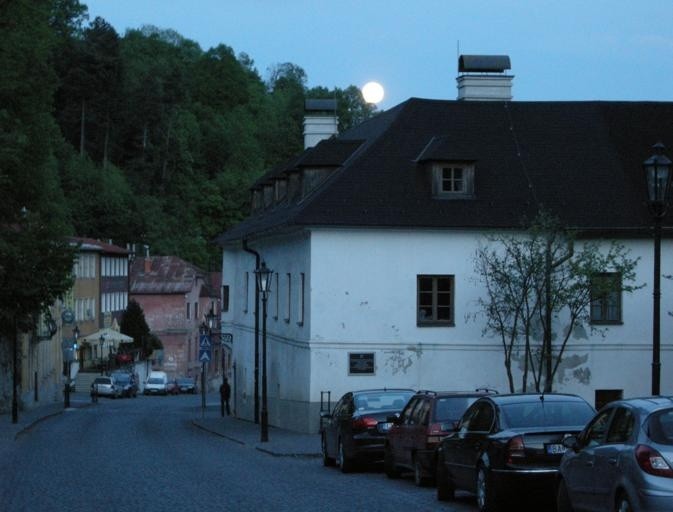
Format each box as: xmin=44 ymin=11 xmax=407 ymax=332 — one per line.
xmin=641 ymin=142 xmax=671 ymax=397
xmin=98 ymin=334 xmax=106 ymax=375
xmin=252 ymin=257 xmax=274 ymax=441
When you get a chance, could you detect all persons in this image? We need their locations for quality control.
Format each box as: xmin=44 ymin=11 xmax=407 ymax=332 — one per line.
xmin=130 ymin=373 xmax=137 ymax=399
xmin=218 ymin=378 xmax=232 ymax=418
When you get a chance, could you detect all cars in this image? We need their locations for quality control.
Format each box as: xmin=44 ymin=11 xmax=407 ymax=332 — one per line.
xmin=553 ymin=396 xmax=673 ymax=512
xmin=89 ymin=371 xmax=197 ymax=399
xmin=319 ymin=388 xmax=419 ymax=474
xmin=383 ymin=388 xmax=510 ymax=487
xmin=434 ymin=391 xmax=599 ymax=512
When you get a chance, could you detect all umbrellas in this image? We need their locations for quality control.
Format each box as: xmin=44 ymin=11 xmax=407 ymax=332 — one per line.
xmin=81 ymin=330 xmax=134 ymax=375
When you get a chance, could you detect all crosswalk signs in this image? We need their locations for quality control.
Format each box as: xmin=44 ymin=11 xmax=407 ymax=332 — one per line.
xmin=199 ymin=335 xmax=212 ymax=347
